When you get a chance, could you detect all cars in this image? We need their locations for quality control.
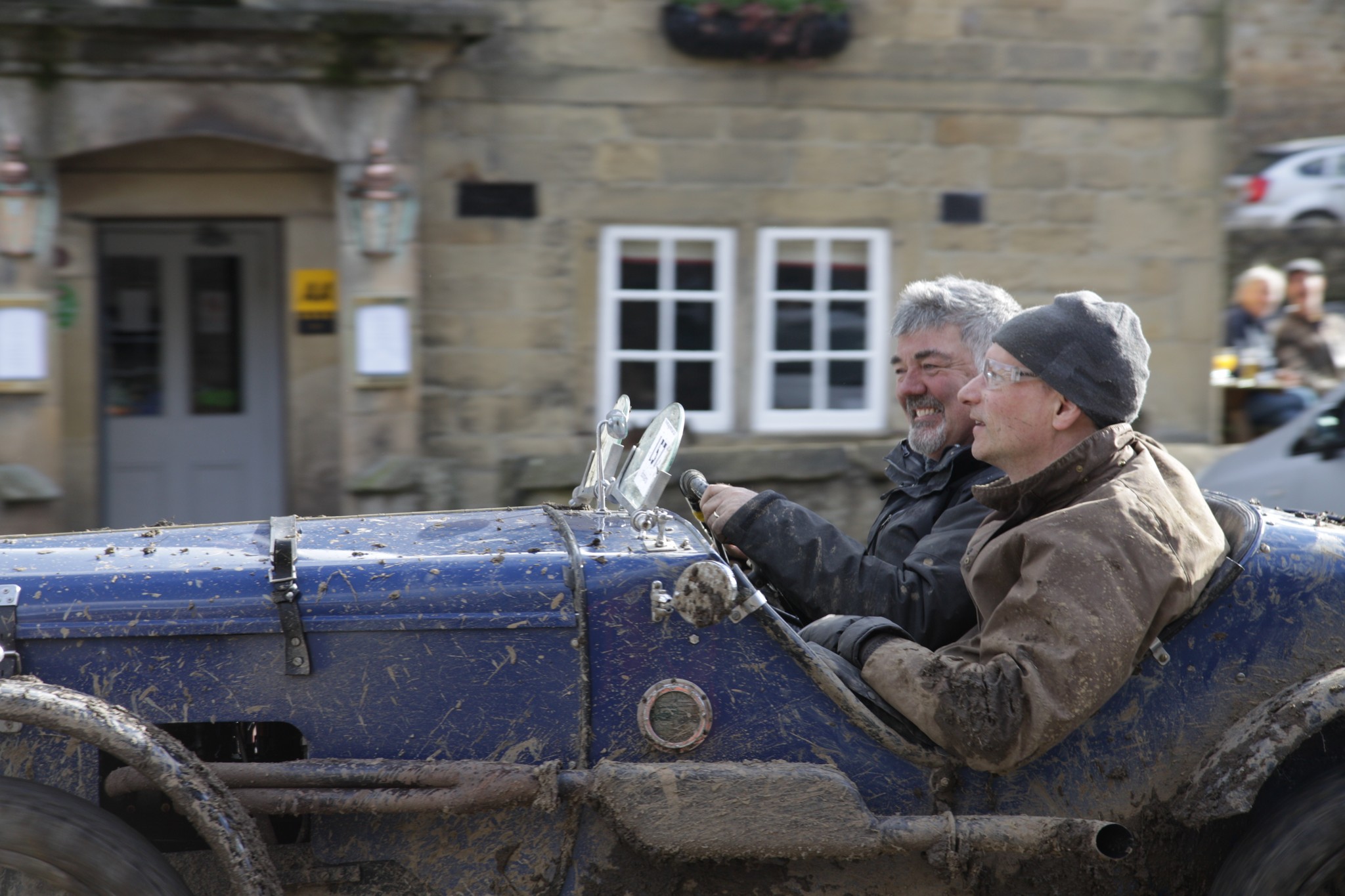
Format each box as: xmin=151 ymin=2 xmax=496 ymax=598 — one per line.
xmin=1228 ymin=134 xmax=1345 ymax=226
xmin=1199 ymin=386 xmax=1345 ymax=528
xmin=1 ymin=394 xmax=1344 ymax=896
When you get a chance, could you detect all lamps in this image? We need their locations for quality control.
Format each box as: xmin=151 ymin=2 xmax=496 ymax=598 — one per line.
xmin=349 ymin=139 xmax=409 ymax=267
xmin=1 ymin=134 xmax=43 ymax=260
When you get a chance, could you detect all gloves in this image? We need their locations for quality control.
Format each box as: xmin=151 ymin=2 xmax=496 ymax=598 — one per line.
xmin=796 ymin=613 xmax=910 ymax=666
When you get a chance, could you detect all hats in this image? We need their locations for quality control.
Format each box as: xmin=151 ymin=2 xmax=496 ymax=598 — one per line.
xmin=992 ymin=290 xmax=1151 ymax=423
xmin=1284 ymin=256 xmax=1325 ymax=275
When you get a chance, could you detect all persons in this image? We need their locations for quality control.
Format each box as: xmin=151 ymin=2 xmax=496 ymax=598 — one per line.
xmin=697 ymin=277 xmax=1026 ymax=637
xmin=1224 ymin=258 xmax=1345 ymax=432
xmin=792 ymin=289 xmax=1228 ymax=775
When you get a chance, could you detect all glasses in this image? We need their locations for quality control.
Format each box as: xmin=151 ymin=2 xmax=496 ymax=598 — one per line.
xmin=982 ymin=357 xmax=1039 ymax=390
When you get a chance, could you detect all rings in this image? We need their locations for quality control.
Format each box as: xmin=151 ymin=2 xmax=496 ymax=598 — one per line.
xmin=713 ymin=510 xmax=719 ymax=518
